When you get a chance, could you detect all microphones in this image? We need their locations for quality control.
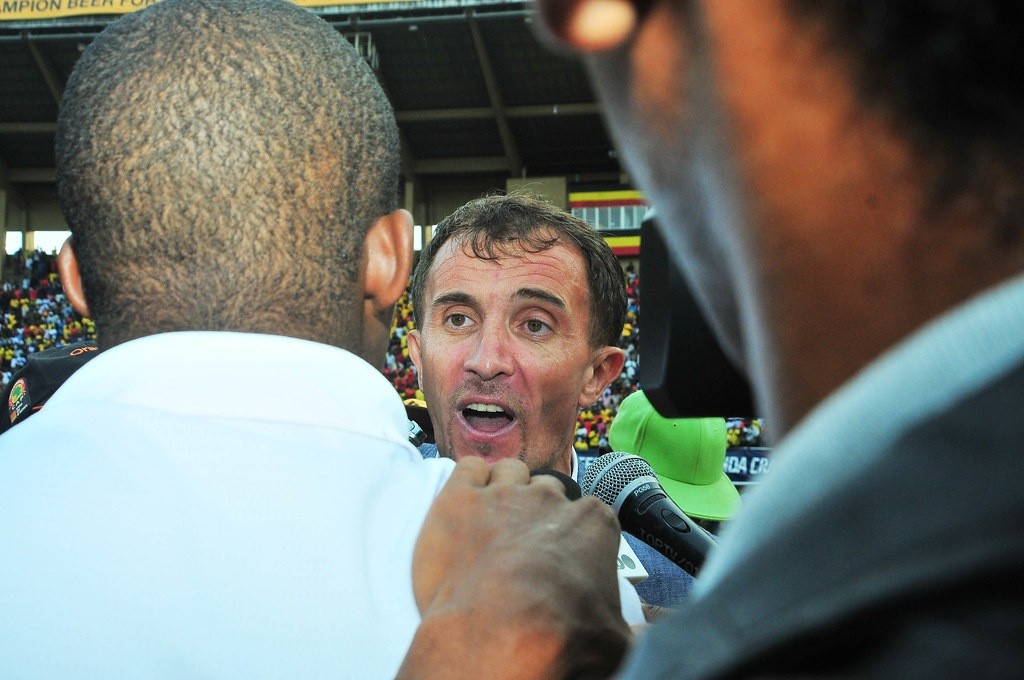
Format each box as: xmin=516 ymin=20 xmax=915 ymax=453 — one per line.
xmin=581 ymin=452 xmax=719 ymax=579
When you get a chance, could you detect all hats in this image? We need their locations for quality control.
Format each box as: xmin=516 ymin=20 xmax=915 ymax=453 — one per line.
xmin=1 ymin=340 xmax=105 ymax=435
xmin=608 ymin=390 xmax=748 ymax=524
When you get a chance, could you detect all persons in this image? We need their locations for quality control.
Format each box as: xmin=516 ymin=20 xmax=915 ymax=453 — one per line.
xmin=0 ymin=0 xmax=639 ymax=680
xmin=0 ymin=239 xmax=100 ymax=435
xmin=396 ymin=0 xmax=1024 ymax=680
xmin=382 ymin=255 xmax=763 ymax=535
xmin=408 ymin=195 xmax=698 ymax=609
